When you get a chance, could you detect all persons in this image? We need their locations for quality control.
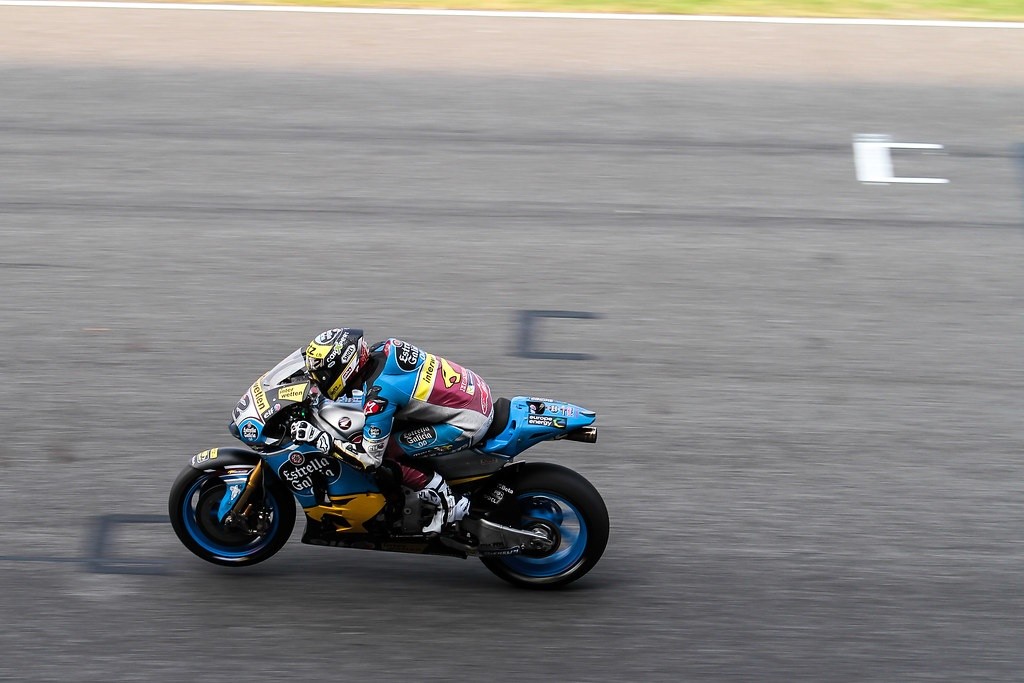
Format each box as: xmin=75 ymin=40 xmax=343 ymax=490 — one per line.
xmin=291 ymin=328 xmax=495 ymax=542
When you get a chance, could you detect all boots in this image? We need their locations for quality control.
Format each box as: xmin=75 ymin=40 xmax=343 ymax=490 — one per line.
xmin=413 ymin=470 xmax=471 ymax=538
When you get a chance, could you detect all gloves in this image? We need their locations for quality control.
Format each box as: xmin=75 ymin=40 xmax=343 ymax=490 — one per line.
xmin=288 ymin=418 xmax=336 ymax=456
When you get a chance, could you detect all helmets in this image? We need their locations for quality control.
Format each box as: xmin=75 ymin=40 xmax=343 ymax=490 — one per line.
xmin=306 ymin=327 xmax=370 ymax=402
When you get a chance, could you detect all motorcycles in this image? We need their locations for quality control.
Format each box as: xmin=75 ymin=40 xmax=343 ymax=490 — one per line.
xmin=168 ymin=346 xmax=610 ymax=590
